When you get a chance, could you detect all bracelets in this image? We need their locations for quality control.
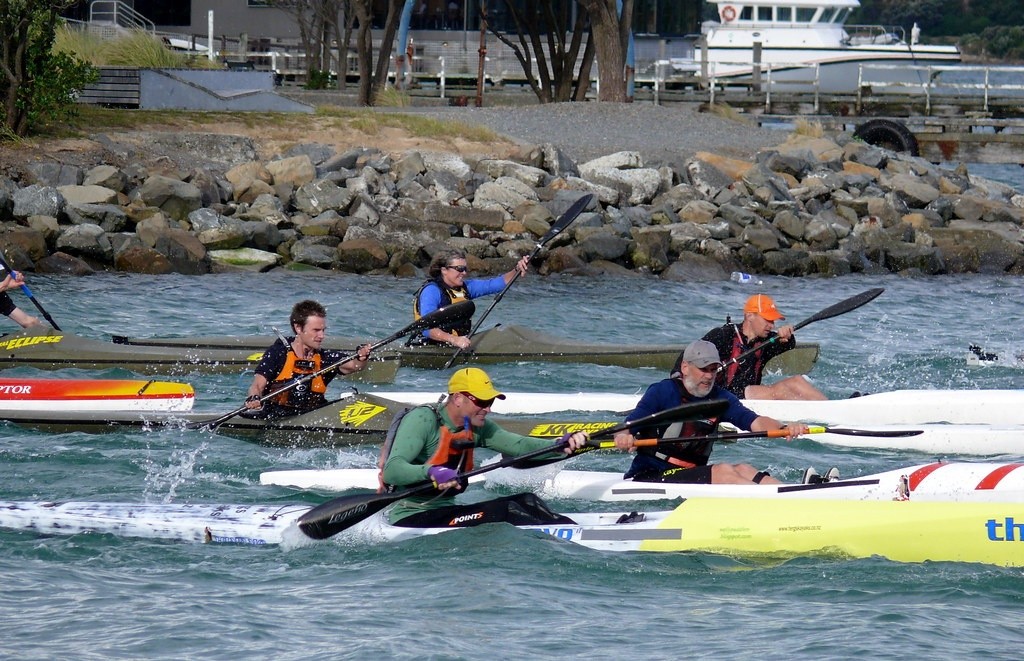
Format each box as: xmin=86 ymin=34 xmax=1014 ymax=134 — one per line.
xmin=515 ymin=265 xmax=518 ymax=272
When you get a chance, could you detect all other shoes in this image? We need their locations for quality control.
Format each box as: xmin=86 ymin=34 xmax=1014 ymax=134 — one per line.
xmin=822 ymin=468 xmax=840 ymax=483
xmin=850 ymin=392 xmax=869 ymax=398
xmin=617 ymin=511 xmax=643 ymax=524
xmin=801 ymin=469 xmax=817 ymax=484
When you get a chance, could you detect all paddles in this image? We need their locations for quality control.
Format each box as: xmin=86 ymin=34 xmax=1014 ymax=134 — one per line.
xmin=296 ymin=396 xmax=733 ymax=542
xmin=0 ymin=249 xmax=62 ymax=331
xmin=443 ymin=192 xmax=594 ymax=368
xmin=503 ymin=424 xmax=925 ymax=470
xmin=720 ymin=285 xmax=887 ymax=373
xmin=183 ymin=300 xmax=476 ymax=432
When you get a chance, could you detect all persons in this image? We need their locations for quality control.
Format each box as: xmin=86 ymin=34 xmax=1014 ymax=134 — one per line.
xmin=0 ymin=263 xmax=45 ymax=329
xmin=239 ymin=299 xmax=372 ymax=421
xmin=378 ymin=367 xmax=646 ymax=528
xmin=614 ymin=341 xmax=840 ymax=485
xmin=406 ymin=248 xmax=529 ymax=349
xmin=669 ymin=294 xmax=869 ymax=401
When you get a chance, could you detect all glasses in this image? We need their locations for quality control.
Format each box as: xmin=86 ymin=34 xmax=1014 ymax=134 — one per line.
xmin=460 ymin=390 xmax=494 ymax=408
xmin=445 ymin=265 xmax=467 ymax=273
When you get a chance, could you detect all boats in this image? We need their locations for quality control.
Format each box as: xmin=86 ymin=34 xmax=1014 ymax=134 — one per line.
xmin=259 ymin=457 xmax=1024 ymax=502
xmin=0 ymin=498 xmax=1024 ymax=572
xmin=0 ymin=378 xmax=194 ymax=412
xmin=648 ymin=0 xmax=960 ymax=96
xmin=334 ymin=388 xmax=1024 ymax=459
xmin=110 ymin=322 xmax=823 ymax=375
xmin=2 ymin=386 xmax=740 ymax=451
xmin=0 ymin=322 xmax=405 ymax=384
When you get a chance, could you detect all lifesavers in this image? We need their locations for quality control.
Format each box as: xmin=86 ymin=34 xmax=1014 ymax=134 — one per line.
xmin=853 ymin=119 xmax=920 ymax=157
xmin=722 ymin=6 xmax=736 ymax=20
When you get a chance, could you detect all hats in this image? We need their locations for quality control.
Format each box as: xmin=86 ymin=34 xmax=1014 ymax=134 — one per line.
xmin=448 ymin=366 xmax=506 ymax=401
xmin=746 ymin=294 xmax=785 ymax=323
xmin=682 ymin=341 xmax=724 ymax=369
